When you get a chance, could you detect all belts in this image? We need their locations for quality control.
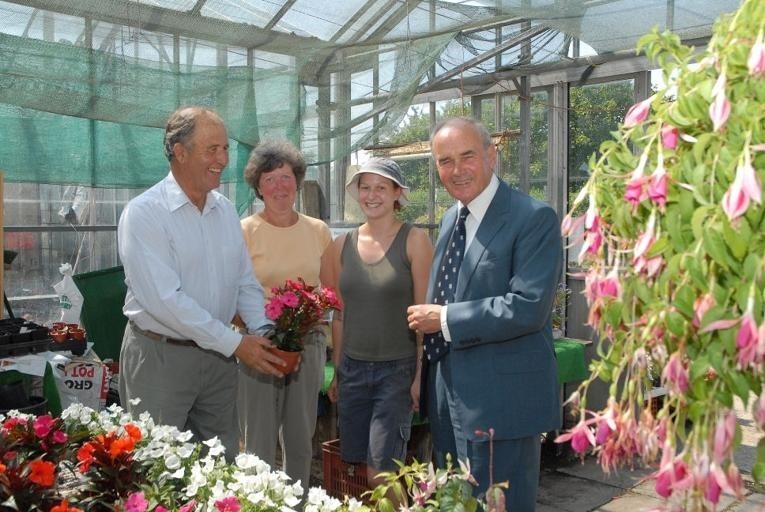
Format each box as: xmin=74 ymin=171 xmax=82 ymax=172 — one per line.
xmin=127 ymin=320 xmax=198 ymax=347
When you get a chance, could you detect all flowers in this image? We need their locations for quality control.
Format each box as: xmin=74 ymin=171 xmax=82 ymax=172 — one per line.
xmin=0 ymin=397 xmax=200 ymax=512
xmin=249 ymin=272 xmax=345 ymax=358
xmin=474 ymin=428 xmax=509 ymax=512
xmin=200 ymin=436 xmax=360 ymax=512
xmin=1 ymin=361 xmax=509 ymax=511
xmin=360 ymin=452 xmax=474 ymax=512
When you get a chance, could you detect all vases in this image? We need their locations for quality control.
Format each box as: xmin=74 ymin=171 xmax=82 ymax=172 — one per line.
xmin=258 ymin=339 xmax=305 ymax=379
xmin=0 ymin=315 xmax=88 ymax=359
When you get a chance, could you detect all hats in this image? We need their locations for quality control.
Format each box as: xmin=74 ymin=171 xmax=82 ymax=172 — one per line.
xmin=344 ymin=156 xmax=412 ymax=210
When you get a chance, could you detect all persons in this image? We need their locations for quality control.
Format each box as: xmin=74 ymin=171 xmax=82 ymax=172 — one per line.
xmin=231 ymin=137 xmax=337 ymax=510
xmin=406 ymin=116 xmax=564 ymax=511
xmin=116 ymin=107 xmax=300 ymax=465
xmin=327 ymin=158 xmax=437 ymax=509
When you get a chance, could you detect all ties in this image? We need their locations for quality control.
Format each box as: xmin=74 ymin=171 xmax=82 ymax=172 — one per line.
xmin=420 ymin=206 xmax=471 ymax=366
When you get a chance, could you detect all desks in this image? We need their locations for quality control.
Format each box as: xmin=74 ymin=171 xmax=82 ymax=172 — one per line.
xmin=549 ymin=330 xmax=594 ymax=461
